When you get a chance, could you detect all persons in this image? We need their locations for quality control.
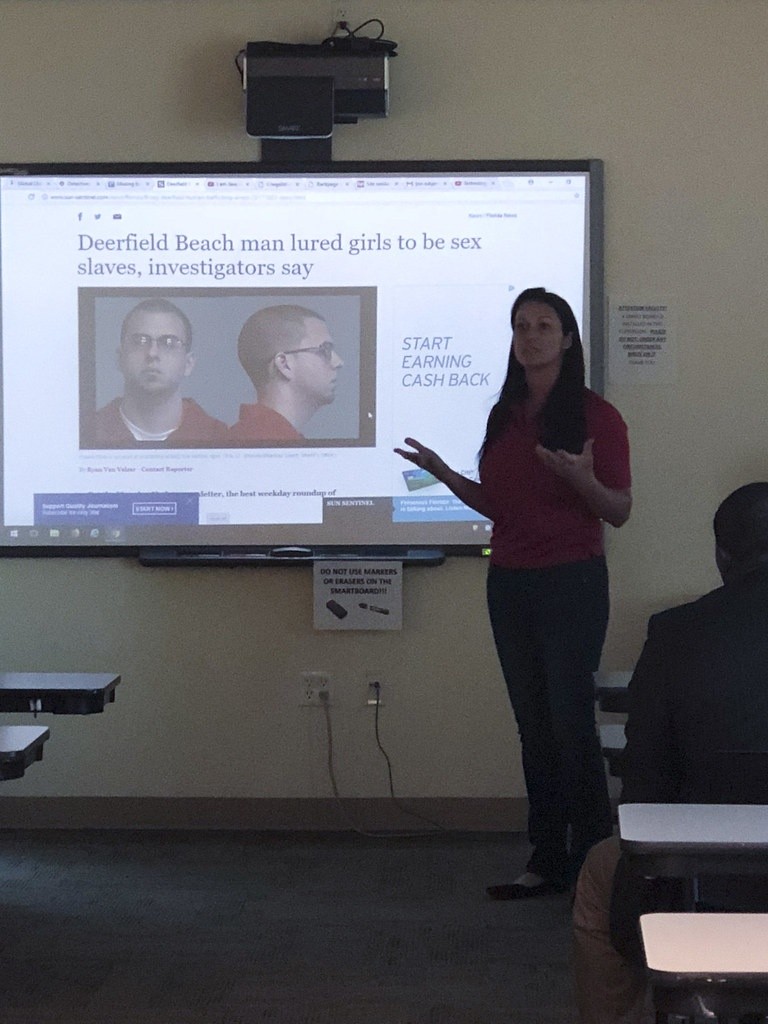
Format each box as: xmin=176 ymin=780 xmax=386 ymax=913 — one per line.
xmin=227 ymin=306 xmax=344 ymax=441
xmin=393 ymin=288 xmax=632 ymax=900
xmin=573 ymin=483 xmax=768 ymax=1024
xmin=96 ymin=299 xmax=228 ymax=448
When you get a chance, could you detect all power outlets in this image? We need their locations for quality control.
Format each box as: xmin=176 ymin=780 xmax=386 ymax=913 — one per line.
xmin=366 ymin=669 xmax=387 ymax=707
xmin=299 ymin=670 xmax=336 ymax=707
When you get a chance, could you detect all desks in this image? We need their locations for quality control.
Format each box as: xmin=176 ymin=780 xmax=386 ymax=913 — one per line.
xmin=617 ymin=802 xmax=768 ymax=911
xmin=0 ymin=724 xmax=51 ymax=782
xmin=0 ymin=672 xmax=122 ymax=720
xmin=599 ymin=724 xmax=627 ymax=777
xmin=592 ymin=670 xmax=634 ymax=714
xmin=637 ymin=910 xmax=768 ymax=1024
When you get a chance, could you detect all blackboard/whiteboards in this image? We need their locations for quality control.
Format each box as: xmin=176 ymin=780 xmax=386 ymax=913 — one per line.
xmin=1 ymin=159 xmax=608 ymax=568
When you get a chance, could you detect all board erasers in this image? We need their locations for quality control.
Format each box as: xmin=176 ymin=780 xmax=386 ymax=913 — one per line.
xmin=272 ymin=547 xmax=312 ymax=558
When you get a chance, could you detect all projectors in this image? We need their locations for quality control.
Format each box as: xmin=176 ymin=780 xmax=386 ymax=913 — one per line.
xmin=246 ymin=44 xmax=390 ymax=139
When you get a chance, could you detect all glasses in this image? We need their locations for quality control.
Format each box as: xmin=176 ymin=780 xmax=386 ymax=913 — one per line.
xmin=122 ymin=333 xmax=187 ymax=353
xmin=269 ymin=341 xmax=334 ymax=363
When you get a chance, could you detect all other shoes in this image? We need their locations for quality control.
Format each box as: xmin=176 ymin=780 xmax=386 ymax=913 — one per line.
xmin=542 ymin=877 xmax=576 ymax=896
xmin=486 ymin=883 xmax=546 ymax=900
